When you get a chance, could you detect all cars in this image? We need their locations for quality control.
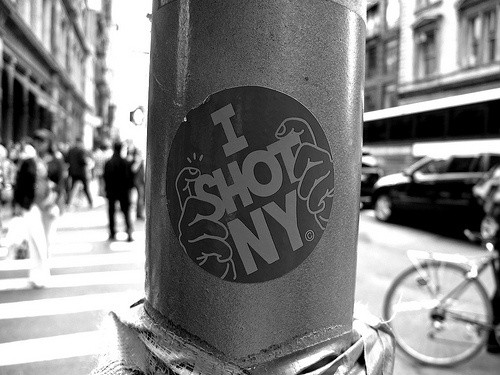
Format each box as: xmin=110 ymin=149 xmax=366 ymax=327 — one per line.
xmin=372 ymin=152 xmax=500 ymax=250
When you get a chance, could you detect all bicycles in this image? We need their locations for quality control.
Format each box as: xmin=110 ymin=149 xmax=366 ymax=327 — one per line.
xmin=381 ymin=231 xmax=500 ymax=368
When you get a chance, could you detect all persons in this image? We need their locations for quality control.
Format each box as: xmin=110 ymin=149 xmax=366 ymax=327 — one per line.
xmin=452 ymin=163 xmax=500 ymax=354
xmin=1 ymin=126 xmax=148 ymax=294
